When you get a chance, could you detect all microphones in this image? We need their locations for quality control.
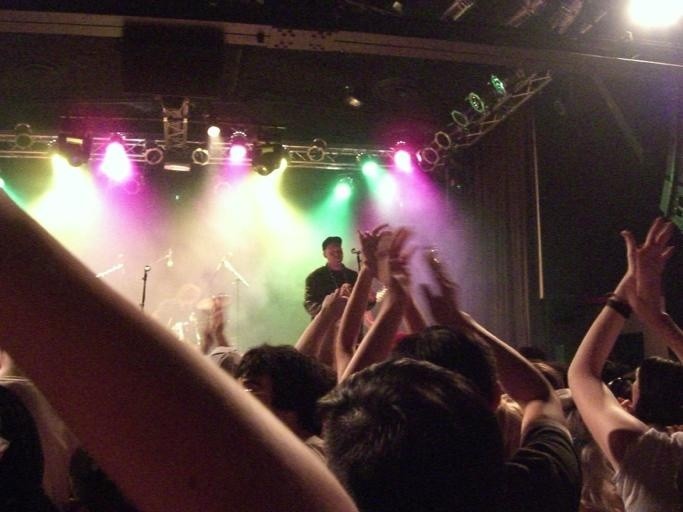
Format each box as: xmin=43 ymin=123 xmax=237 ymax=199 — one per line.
xmin=143 ymin=265 xmax=151 ymax=281
xmin=166 ymin=251 xmax=174 ymax=269
xmin=351 ymin=248 xmax=361 ymax=254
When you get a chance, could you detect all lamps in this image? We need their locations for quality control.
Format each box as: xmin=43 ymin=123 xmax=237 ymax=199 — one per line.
xmin=142 ymin=116 xmax=330 ymax=178
xmin=11 ymin=120 xmax=93 ymax=168
xmin=441 ymin=1 xmax=584 ymax=38
xmin=420 ymin=66 xmax=517 ymax=167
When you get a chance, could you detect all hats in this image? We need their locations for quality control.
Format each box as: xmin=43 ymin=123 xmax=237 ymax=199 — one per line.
xmin=322 ymin=237 xmax=342 ymax=251
